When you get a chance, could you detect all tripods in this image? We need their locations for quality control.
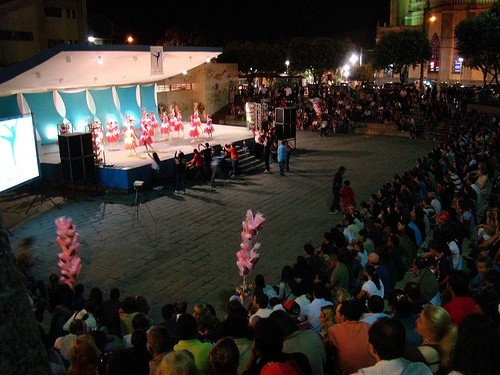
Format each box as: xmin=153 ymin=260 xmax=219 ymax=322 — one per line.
xmin=132 ymin=185 xmax=158 ymax=228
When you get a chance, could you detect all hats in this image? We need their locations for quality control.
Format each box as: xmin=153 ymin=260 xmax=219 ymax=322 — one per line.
xmin=434 ymin=212 xmax=450 ymax=220
xmin=367 ymin=253 xmax=381 ymax=264
xmin=282 ymin=300 xmax=301 ymax=314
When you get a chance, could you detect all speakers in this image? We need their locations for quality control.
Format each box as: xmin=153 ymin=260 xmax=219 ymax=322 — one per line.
xmin=58 ymin=132 xmax=94 ymax=182
xmin=274 ymin=107 xmax=295 ymax=140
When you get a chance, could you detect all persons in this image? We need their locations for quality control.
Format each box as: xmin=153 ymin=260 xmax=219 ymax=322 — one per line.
xmin=57 ymin=106 xmax=215 ymax=154
xmin=148 ymin=143 xmax=239 ymax=195
xmin=325 ymin=109 xmax=500 ymax=375
xmin=229 ymin=80 xmax=469 ymax=139
xmin=254 ymin=123 xmax=292 ymax=176
xmin=29 ymin=243 xmax=333 ymax=375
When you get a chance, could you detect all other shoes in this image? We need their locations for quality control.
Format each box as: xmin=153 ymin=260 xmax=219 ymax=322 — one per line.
xmin=329 ymin=209 xmax=340 ymax=214
xmin=230 ymin=175 xmax=236 ymax=177
xmin=264 ymin=169 xmax=271 ymax=174
xmin=153 ymin=186 xmax=163 ymax=190
xmin=174 ymin=190 xmax=186 ymax=194
